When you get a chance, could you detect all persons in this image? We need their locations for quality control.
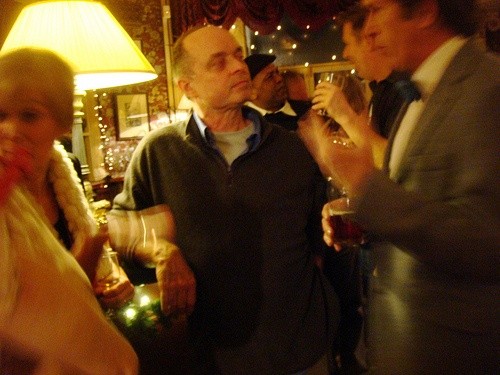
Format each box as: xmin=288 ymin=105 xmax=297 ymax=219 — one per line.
xmin=243 ymin=52 xmax=316 ymax=132
xmin=0 ymin=48 xmax=142 ymax=374
xmin=108 ymin=25 xmax=331 ymax=374
xmin=296 ymin=0 xmax=500 ymax=375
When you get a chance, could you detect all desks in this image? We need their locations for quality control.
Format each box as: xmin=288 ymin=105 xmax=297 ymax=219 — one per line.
xmin=89 ymin=171 xmax=127 ymax=208
xmin=110 ymin=282 xmax=190 ymax=375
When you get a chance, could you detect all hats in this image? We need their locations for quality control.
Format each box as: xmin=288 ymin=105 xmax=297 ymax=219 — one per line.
xmin=244 ymin=54 xmax=277 ymax=80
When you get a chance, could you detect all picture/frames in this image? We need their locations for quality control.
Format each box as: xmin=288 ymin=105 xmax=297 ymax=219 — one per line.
xmin=111 ymin=93 xmax=151 ymax=141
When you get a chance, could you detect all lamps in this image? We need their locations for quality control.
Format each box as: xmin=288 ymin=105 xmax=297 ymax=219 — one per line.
xmin=0 ymin=2 xmax=159 ymax=91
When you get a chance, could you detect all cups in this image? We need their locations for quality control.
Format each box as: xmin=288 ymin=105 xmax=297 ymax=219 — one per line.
xmin=317 ymin=73 xmax=346 ymax=115
xmin=327 ymin=185 xmax=366 ymax=246
xmin=96 ymin=253 xmax=119 ymax=286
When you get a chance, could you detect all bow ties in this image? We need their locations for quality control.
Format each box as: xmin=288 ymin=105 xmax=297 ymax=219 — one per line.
xmin=395 ymin=80 xmax=419 ymax=100
